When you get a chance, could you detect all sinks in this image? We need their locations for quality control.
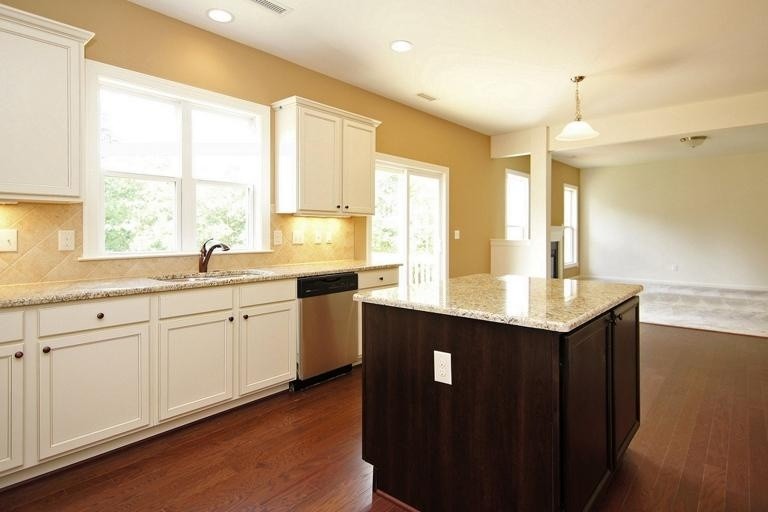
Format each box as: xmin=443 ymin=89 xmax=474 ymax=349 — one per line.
xmin=145 ymin=270 xmax=274 ymax=282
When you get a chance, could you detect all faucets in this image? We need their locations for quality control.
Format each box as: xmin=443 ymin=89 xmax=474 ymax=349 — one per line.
xmin=198 ymin=238 xmax=230 ymax=272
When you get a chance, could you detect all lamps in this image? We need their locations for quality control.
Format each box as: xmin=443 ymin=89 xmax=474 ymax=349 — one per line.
xmin=554 ymin=76 xmax=600 ymax=141
xmin=679 ymin=135 xmax=707 ymax=148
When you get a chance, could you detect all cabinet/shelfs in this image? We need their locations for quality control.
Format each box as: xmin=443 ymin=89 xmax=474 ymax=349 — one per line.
xmin=270 ymin=95 xmax=382 ymax=215
xmin=0 ymin=292 xmax=154 ymax=489
xmin=0 ymin=4 xmax=95 ymax=204
xmin=558 ymin=294 xmax=641 ymax=512
xmin=154 ymin=276 xmax=298 ymax=436
xmin=356 ymin=268 xmax=400 ymax=365
